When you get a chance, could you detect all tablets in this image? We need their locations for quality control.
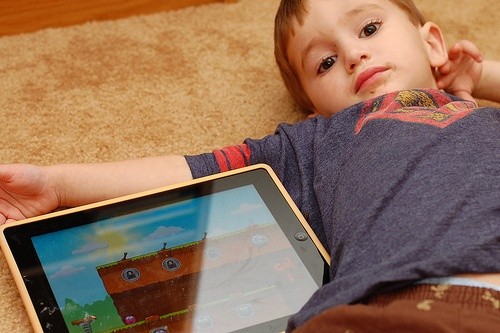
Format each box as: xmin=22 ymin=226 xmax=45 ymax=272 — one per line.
xmin=0 ymin=163 xmax=331 ymax=333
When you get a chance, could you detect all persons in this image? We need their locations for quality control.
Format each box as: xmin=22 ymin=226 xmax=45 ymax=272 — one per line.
xmin=0 ymin=0 xmax=500 ymax=333
